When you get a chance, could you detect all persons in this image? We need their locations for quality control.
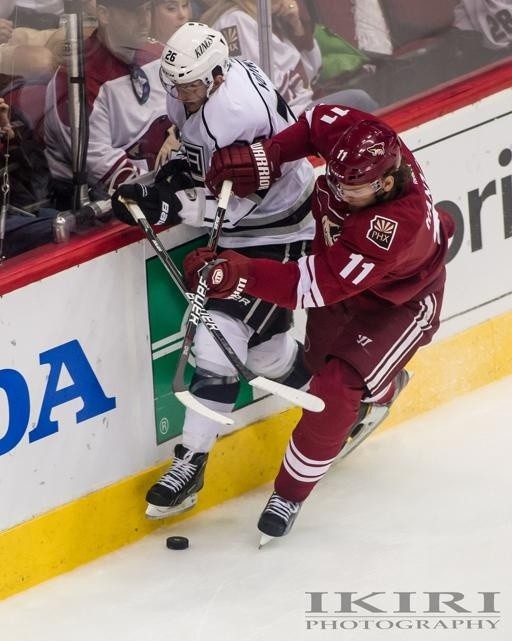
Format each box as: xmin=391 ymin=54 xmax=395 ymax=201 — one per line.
xmin=112 ymin=22 xmax=318 ymax=508
xmin=145 ymin=102 xmax=455 ymax=535
xmin=1 ymin=0 xmax=512 ymax=266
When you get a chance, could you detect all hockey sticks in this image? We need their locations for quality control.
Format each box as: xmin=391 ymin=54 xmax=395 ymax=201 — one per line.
xmin=124 ymin=201 xmax=326 ymax=412
xmin=172 ymin=179 xmax=235 ymax=426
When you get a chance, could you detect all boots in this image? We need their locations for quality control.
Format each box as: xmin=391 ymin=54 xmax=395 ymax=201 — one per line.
xmin=340 ymin=399 xmax=372 ymax=452
xmin=257 ymin=490 xmax=301 ymax=536
xmin=145 ymin=445 xmax=208 ymax=507
xmin=380 ymin=369 xmax=409 ymax=410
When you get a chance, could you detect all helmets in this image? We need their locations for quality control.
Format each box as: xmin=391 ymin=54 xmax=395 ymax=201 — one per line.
xmin=157 ymin=21 xmax=230 ymax=103
xmin=324 ymin=119 xmax=401 ymax=203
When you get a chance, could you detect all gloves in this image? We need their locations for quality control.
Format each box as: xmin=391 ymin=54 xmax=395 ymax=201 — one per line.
xmin=111 ymin=184 xmax=182 ymax=228
xmin=205 ymin=140 xmax=282 ymax=198
xmin=183 ymin=248 xmax=256 ymax=301
xmin=154 ymin=159 xmax=194 ymax=194
